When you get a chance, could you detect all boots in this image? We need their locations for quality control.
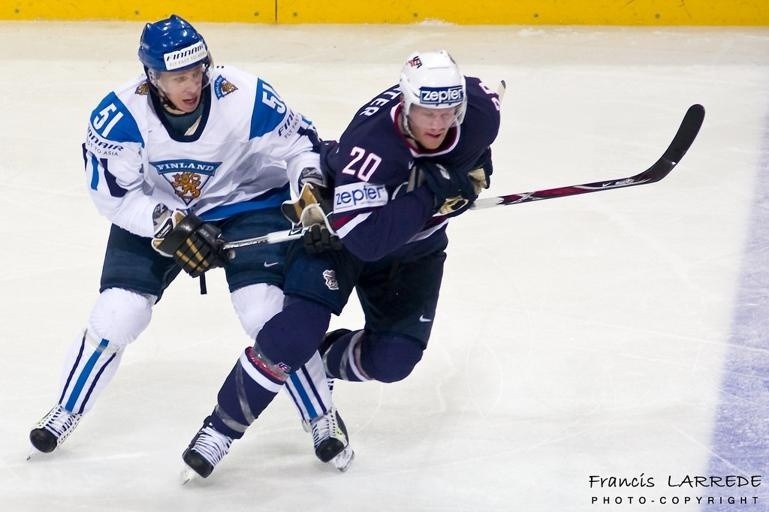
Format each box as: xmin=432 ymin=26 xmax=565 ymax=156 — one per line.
xmin=309 ymin=405 xmax=349 ymax=463
xmin=317 ymin=330 xmax=340 ymax=392
xmin=182 ymin=416 xmax=233 ymax=478
xmin=30 ymin=403 xmax=82 ymax=452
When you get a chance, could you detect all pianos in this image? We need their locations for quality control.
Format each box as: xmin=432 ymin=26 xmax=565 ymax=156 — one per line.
xmin=218 ymin=104 xmax=704 ymax=248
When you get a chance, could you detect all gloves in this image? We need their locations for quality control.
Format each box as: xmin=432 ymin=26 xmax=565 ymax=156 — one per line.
xmin=281 ymin=183 xmax=344 ymax=256
xmin=423 ymin=165 xmax=490 ymax=217
xmin=151 ymin=203 xmax=229 ymax=278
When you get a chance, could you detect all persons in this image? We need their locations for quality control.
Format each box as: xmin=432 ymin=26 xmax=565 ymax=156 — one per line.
xmin=179 ymin=50 xmax=502 ymax=485
xmin=25 ymin=13 xmax=355 ymax=472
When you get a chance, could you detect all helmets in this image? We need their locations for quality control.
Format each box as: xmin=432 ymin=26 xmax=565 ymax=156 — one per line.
xmin=396 ymin=50 xmax=468 ymax=130
xmin=138 ymin=14 xmax=211 ymax=72
